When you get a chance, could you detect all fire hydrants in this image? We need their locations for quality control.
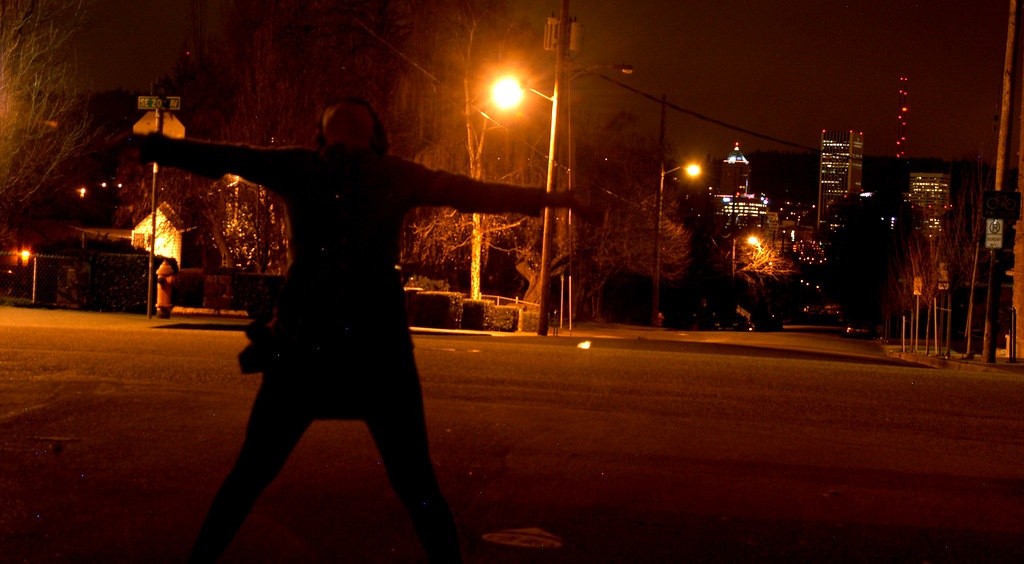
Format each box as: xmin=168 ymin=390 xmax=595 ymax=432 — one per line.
xmin=154 ymin=258 xmax=179 ymax=318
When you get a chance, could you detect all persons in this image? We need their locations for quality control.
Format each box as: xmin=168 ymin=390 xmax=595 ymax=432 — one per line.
xmin=107 ymin=95 xmax=579 ymax=564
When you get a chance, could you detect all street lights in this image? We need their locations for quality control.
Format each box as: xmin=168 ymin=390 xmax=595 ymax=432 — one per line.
xmin=565 ymin=63 xmax=634 ymax=333
xmin=488 ymin=75 xmax=561 ymax=336
xmin=648 ymin=164 xmax=704 ymax=328
